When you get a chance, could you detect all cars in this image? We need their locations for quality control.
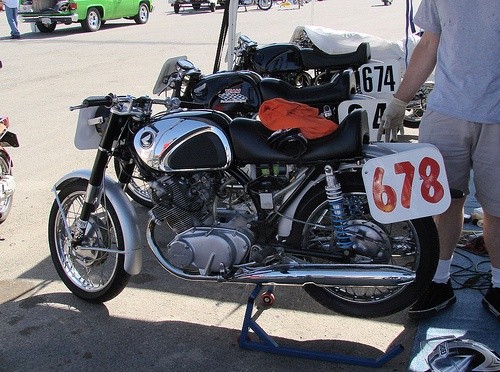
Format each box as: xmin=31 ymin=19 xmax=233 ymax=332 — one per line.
xmin=17 ymin=0 xmax=154 ymax=34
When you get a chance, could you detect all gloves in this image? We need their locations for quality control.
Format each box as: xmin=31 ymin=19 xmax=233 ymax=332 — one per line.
xmin=377 ymin=96 xmax=409 ymax=143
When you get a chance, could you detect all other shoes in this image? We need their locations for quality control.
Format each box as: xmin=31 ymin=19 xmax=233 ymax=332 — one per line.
xmin=9 ymin=35 xmax=20 ymax=39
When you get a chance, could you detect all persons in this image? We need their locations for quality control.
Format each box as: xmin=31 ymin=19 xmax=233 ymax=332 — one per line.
xmin=376 ymin=0 xmax=500 ymax=320
xmin=1 ymin=0 xmax=21 ymax=39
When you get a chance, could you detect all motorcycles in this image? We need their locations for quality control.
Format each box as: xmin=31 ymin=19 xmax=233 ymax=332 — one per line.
xmin=113 ymin=28 xmax=435 ymax=262
xmin=48 ymin=94 xmax=441 ymax=346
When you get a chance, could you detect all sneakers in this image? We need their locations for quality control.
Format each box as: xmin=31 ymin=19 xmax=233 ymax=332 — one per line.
xmin=408 ymin=278 xmax=456 ymax=318
xmin=481 ymin=283 xmax=500 ymax=321
